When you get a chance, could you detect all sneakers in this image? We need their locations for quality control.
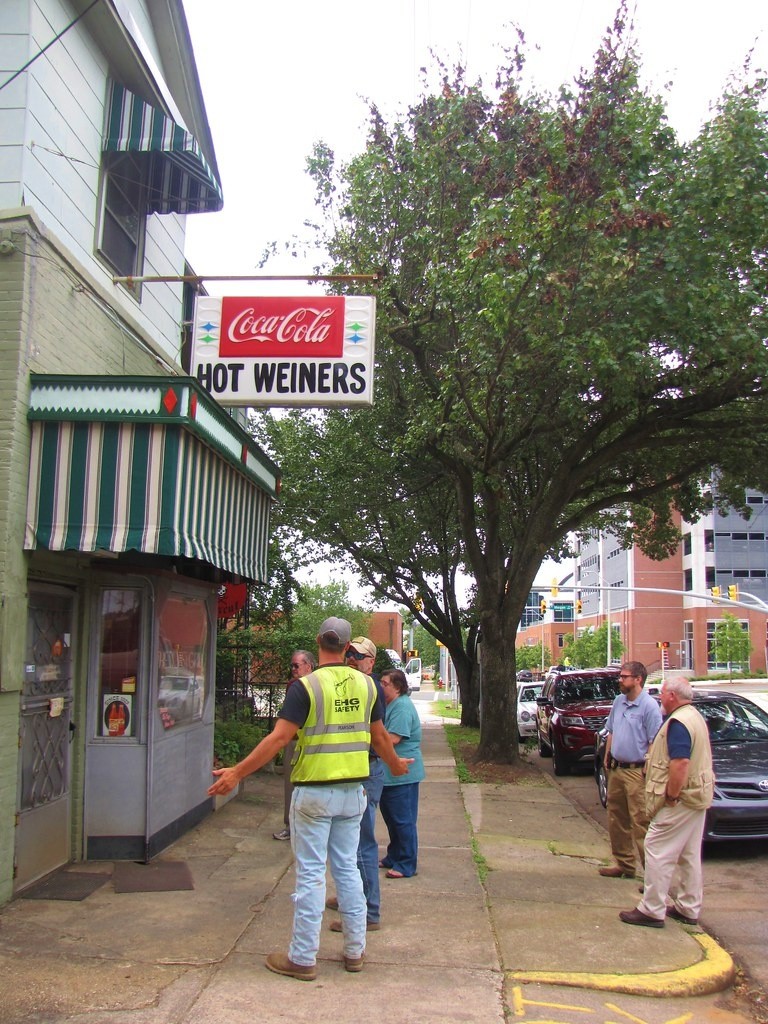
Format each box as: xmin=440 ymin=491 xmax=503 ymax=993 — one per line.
xmin=345 ymin=952 xmax=366 ymax=972
xmin=620 ymin=907 xmax=666 ymax=928
xmin=267 ymin=953 xmax=316 ymax=981
xmin=666 ymin=905 xmax=698 ymax=925
xmin=599 ymin=866 xmax=636 ymax=878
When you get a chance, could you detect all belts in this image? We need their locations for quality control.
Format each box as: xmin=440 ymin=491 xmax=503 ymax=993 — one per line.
xmin=611 ymin=759 xmax=645 ymax=770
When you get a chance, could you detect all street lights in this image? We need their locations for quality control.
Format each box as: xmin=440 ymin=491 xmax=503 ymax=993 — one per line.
xmin=583 ymin=571 xmax=612 ymax=665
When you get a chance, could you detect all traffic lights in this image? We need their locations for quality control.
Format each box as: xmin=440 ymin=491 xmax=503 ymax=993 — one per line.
xmin=578 ymin=600 xmax=582 ymax=613
xmin=656 ymin=641 xmax=660 ymax=648
xmin=663 ymin=642 xmax=669 ymax=647
xmin=711 ymin=587 xmax=719 ymax=603
xmin=542 ymin=601 xmax=546 ymax=614
xmin=727 ymin=585 xmax=736 ymax=601
xmin=408 ymin=651 xmax=415 ymax=657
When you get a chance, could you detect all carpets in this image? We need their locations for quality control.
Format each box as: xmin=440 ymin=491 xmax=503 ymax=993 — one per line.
xmin=20 ymin=870 xmax=111 ymax=902
xmin=111 ymin=860 xmax=196 ymax=894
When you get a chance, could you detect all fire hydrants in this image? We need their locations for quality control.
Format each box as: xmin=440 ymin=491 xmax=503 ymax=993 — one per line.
xmin=437 ymin=676 xmax=445 ymax=688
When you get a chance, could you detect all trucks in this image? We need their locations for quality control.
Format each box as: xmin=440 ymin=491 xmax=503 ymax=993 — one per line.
xmin=383 ymin=648 xmax=422 ymax=697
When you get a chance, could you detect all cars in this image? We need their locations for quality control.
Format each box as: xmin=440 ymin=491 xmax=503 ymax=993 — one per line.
xmin=593 ymin=690 xmax=768 ymax=844
xmin=517 ymin=681 xmax=546 ymax=743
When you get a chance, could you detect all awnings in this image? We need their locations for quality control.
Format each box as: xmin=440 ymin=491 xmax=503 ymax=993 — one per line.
xmin=24 ymin=372 xmax=283 ymax=585
xmin=102 ymin=77 xmax=225 ymax=217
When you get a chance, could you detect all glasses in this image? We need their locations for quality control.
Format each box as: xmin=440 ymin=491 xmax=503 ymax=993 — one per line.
xmin=290 ymin=662 xmax=306 ymax=669
xmin=345 ymin=651 xmax=372 ymax=660
xmin=620 ymin=673 xmax=636 ymax=679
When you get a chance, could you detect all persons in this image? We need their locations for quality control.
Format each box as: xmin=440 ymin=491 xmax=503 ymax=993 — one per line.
xmin=619 ymin=677 xmax=714 ymax=928
xmin=598 ymin=661 xmax=664 ymax=893
xmin=325 ymin=635 xmax=385 ymax=933
xmin=207 ymin=618 xmax=414 ymax=981
xmin=272 ymin=649 xmax=316 ymax=840
xmin=379 ymin=668 xmax=426 ymax=879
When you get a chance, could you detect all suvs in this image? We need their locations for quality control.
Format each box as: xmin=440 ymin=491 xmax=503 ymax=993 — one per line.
xmin=517 ymin=670 xmax=533 ymax=682
xmin=535 ymin=664 xmax=659 ymax=776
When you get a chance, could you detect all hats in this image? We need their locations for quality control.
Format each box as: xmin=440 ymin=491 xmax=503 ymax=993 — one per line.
xmin=319 ymin=616 xmax=352 ymax=645
xmin=351 ymin=636 xmax=377 ymax=657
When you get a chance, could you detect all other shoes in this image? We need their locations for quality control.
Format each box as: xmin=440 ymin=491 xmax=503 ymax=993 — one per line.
xmin=377 ymin=860 xmax=386 ymax=868
xmin=327 ymin=897 xmax=339 ymax=910
xmin=330 ymin=921 xmax=381 ymax=931
xmin=386 ymin=868 xmax=419 ymax=878
xmin=273 ymin=830 xmax=290 ymax=840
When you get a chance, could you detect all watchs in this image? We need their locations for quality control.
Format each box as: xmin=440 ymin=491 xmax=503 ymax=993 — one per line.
xmin=665 ymin=793 xmax=678 ymax=802
